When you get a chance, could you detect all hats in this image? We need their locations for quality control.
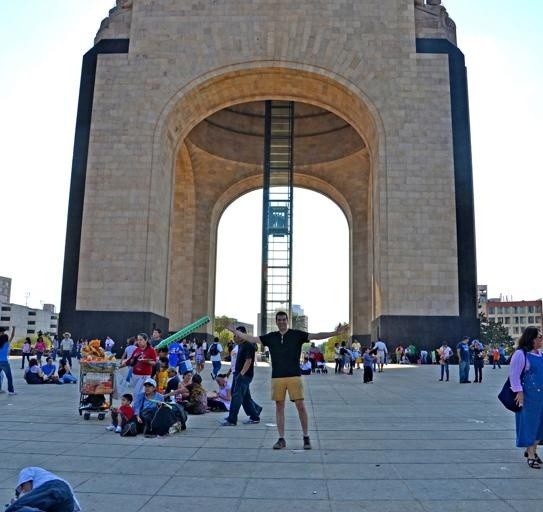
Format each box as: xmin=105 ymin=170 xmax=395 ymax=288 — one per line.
xmin=464 ymin=335 xmax=471 ymax=339
xmin=62 ymin=331 xmax=72 ymax=338
xmin=142 ymin=378 xmax=158 ymax=388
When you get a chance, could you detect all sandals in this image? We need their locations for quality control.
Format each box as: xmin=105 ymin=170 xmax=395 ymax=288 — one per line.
xmin=524 ymin=449 xmax=543 ymax=469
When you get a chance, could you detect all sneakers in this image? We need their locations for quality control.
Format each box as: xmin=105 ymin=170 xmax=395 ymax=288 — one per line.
xmin=302 ymin=435 xmax=312 ymax=449
xmin=216 ymin=417 xmax=237 ymax=426
xmin=241 ymin=417 xmax=261 ymax=425
xmin=104 ymin=425 xmax=123 ymax=433
xmin=272 ymin=437 xmax=286 ymax=449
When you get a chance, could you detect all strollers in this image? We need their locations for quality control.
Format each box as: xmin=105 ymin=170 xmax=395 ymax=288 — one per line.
xmin=311 ymin=353 xmax=327 ymax=374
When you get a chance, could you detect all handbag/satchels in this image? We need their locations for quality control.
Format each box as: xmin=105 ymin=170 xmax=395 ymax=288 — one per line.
xmin=497 ymin=376 xmax=523 ymax=412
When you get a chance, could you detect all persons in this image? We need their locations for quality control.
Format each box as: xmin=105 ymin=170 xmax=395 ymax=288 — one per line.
xmin=300 ymin=337 xmax=515 ymax=383
xmin=222 ymin=312 xmax=350 ymax=449
xmin=5 ymin=467 xmax=81 ymax=512
xmin=1 ymin=326 xmax=76 ymax=395
xmin=77 ymin=326 xmax=262 ymax=437
xmin=509 ymin=327 xmax=543 ymax=469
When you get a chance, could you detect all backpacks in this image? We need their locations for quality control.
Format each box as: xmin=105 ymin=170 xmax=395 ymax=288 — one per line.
xmin=210 ymin=343 xmax=219 ymax=355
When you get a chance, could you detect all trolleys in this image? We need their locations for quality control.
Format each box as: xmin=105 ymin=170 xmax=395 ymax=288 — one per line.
xmin=78 ymin=361 xmax=117 ymax=422
